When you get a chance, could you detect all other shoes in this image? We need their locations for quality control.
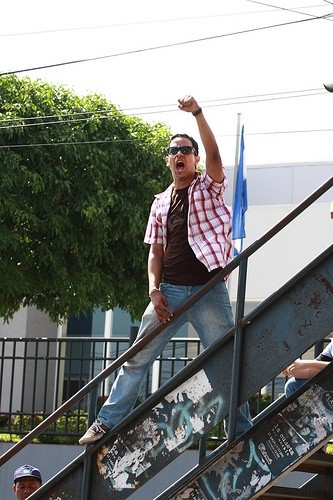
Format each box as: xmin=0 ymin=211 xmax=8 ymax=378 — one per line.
xmin=78 ymin=420 xmax=105 ymax=445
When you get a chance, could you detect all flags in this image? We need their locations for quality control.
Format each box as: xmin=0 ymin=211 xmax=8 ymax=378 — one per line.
xmin=231 ymin=123 xmax=248 ymax=258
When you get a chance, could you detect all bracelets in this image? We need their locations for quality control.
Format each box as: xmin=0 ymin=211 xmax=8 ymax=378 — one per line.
xmin=148 ymin=287 xmax=159 ymax=296
xmin=193 ymin=108 xmax=202 ymax=116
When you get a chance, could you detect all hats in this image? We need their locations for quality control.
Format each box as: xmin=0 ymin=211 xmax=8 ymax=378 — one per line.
xmin=13 ymin=464 xmax=41 ymax=485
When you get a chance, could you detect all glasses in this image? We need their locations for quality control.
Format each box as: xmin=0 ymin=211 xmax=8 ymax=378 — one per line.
xmin=166 ymin=146 xmax=197 ymax=155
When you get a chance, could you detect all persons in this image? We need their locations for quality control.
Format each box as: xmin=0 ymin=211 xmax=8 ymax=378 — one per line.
xmin=78 ymin=94 xmax=252 ymax=444
xmin=281 ymin=338 xmax=333 ymax=399
xmin=13 ymin=464 xmax=43 ymax=500
